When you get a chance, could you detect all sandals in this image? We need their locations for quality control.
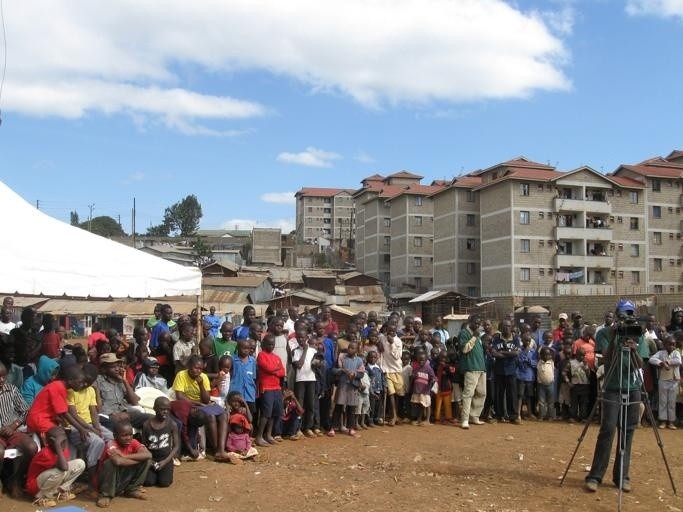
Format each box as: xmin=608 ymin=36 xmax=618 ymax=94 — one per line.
xmin=356 ymin=418 xmax=459 ymax=431
xmin=256 ymin=426 xmax=356 ymax=447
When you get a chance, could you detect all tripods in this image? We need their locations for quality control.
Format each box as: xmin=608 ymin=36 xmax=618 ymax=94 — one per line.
xmin=560 ymin=344 xmax=677 ymax=511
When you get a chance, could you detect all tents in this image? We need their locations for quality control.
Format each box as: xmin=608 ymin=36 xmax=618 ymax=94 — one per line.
xmin=0 ymin=182 xmax=202 ymax=347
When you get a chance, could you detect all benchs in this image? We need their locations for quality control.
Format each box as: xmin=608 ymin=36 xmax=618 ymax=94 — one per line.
xmin=3 ymin=448 xmax=23 ymax=498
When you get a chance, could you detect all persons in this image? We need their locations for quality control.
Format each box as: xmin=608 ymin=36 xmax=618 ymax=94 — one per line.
xmin=643 ymin=307 xmax=683 ymax=430
xmin=584 ymin=299 xmax=649 ymax=491
xmin=27 ymin=426 xmax=86 ymax=507
xmin=1 ymin=299 xmax=683 ymax=486
xmin=92 ymin=423 xmax=152 ymax=507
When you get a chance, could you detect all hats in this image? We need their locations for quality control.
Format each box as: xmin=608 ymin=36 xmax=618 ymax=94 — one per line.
xmin=617 ymin=302 xmax=635 ymax=311
xmin=672 ymin=307 xmax=683 ymax=317
xmin=572 ymin=312 xmax=582 ymax=320
xmin=99 ymin=352 xmax=125 ymax=364
xmin=413 ymin=317 xmax=422 ymax=324
xmin=559 ymin=312 xmax=568 ymax=320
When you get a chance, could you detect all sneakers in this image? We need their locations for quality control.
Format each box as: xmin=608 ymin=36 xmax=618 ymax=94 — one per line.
xmin=668 ymin=424 xmax=676 ymax=429
xmin=585 ymin=482 xmax=598 ymax=492
xmin=58 ymin=492 xmax=76 ymax=500
xmin=623 ymin=484 xmax=631 ymax=491
xmin=172 ymin=457 xmax=181 ymax=466
xmin=470 ymin=417 xmax=484 ymax=425
xmin=181 ymin=453 xmax=204 ymax=461
xmin=488 ymin=414 xmax=587 ymax=425
xmin=659 ymin=424 xmax=666 ymax=429
xmin=35 ymin=498 xmax=57 ymax=507
xmin=461 ymin=420 xmax=469 ymax=428
